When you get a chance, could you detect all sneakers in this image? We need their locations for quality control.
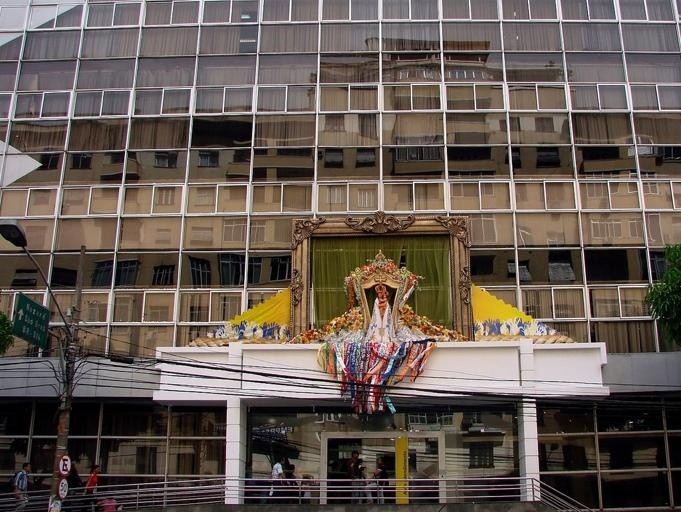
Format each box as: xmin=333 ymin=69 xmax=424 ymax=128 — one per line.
xmin=351 ymin=499 xmax=374 ymax=506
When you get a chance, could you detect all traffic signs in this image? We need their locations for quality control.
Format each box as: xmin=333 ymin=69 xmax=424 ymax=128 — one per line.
xmin=13 ymin=291 xmax=51 ymax=350
xmin=59 ymin=479 xmax=68 ymax=499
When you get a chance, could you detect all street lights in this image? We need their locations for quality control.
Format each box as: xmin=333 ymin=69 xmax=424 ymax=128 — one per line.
xmin=0 ymin=220 xmax=85 ymax=512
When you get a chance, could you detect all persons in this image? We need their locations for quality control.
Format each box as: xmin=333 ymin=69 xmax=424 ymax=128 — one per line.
xmin=379 ymin=290 xmax=388 ymax=315
xmin=83 ymin=464 xmax=99 ymax=510
xmin=269 ymin=455 xmax=287 ymax=498
xmin=352 ymin=458 xmax=366 ymax=504
xmin=347 ymin=449 xmax=361 ymax=501
xmin=378 ymin=291 xmax=385 ymax=303
xmin=364 ymin=455 xmax=387 ymax=506
xmin=14 ymin=461 xmax=32 ymax=512
xmin=96 ymin=491 xmax=120 ymax=512
